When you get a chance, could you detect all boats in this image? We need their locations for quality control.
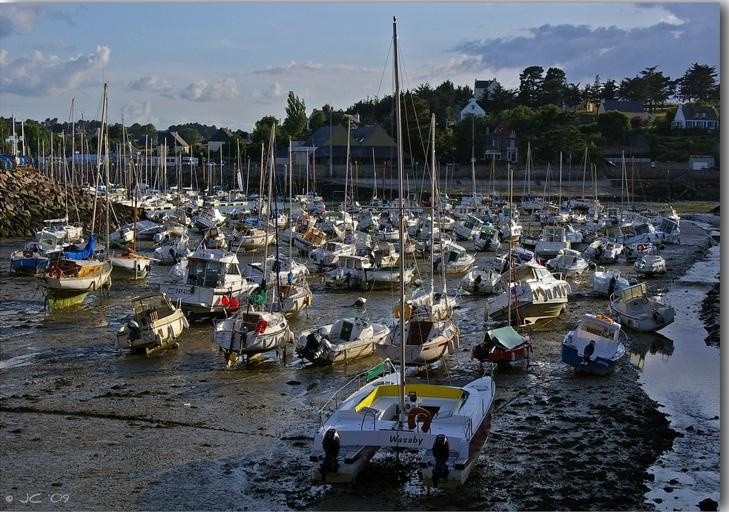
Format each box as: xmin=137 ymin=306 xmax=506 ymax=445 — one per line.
xmin=0 ymin=79 xmax=681 ymax=377
xmin=309 ymin=16 xmax=498 ymax=491
